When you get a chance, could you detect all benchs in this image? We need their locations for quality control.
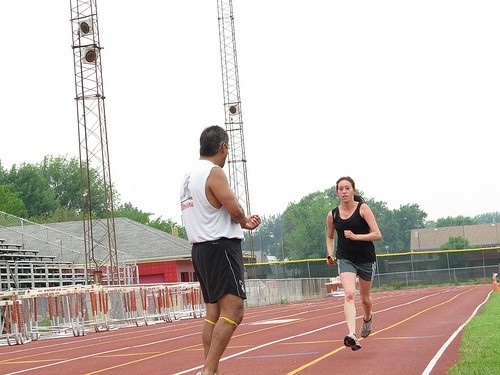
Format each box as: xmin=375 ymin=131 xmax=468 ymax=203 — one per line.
xmin=0 ymin=238 xmax=93 ymax=291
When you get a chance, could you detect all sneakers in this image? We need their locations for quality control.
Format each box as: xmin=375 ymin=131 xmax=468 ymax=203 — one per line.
xmin=344 ymin=333 xmax=362 ymax=351
xmin=361 ymin=314 xmax=373 ymax=338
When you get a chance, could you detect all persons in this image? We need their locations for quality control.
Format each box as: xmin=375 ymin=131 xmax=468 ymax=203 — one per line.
xmin=326 ymin=177 xmax=382 ymax=351
xmin=179 ymin=125 xmax=261 ymax=375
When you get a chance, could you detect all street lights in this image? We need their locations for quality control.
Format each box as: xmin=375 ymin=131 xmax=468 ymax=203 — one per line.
xmin=491 ymin=219 xmax=499 ymax=246
xmin=404 ymin=217 xmax=438 ymax=264
